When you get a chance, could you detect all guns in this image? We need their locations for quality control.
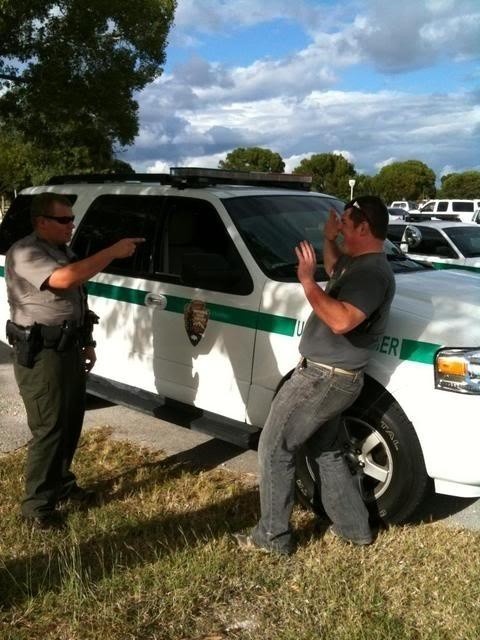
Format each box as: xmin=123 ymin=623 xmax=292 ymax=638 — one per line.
xmin=6 ymin=320 xmax=37 ymax=369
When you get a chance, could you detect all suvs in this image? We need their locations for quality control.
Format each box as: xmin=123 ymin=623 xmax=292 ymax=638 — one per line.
xmin=0 ymin=164 xmax=478 ymax=525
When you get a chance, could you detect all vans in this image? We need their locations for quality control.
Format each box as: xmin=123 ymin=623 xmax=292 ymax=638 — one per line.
xmin=372 ymin=195 xmax=480 ymax=270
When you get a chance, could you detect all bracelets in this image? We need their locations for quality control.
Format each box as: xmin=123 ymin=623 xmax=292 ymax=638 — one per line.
xmin=84 ymin=341 xmax=97 ymax=349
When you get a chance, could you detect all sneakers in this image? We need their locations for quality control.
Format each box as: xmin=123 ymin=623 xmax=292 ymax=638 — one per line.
xmin=328 ymin=524 xmax=373 ymax=546
xmin=63 ymin=482 xmax=96 ymax=507
xmin=21 ymin=505 xmax=67 ymax=532
xmin=235 ymin=533 xmax=275 ymax=553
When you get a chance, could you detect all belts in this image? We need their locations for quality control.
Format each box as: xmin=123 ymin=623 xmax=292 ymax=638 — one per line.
xmin=299 ymin=355 xmax=357 ymax=376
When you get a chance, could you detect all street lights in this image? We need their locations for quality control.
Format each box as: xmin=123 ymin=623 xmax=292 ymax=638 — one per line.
xmin=347 ymin=179 xmax=356 ymax=201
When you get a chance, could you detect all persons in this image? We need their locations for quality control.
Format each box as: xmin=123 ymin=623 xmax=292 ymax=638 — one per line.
xmin=234 ymin=193 xmax=397 ymax=555
xmin=3 ymin=192 xmax=148 ymax=530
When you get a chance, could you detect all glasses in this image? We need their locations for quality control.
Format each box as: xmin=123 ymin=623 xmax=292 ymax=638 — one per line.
xmin=344 ymin=199 xmax=377 ymax=235
xmin=37 ymin=213 xmax=76 ymax=224
